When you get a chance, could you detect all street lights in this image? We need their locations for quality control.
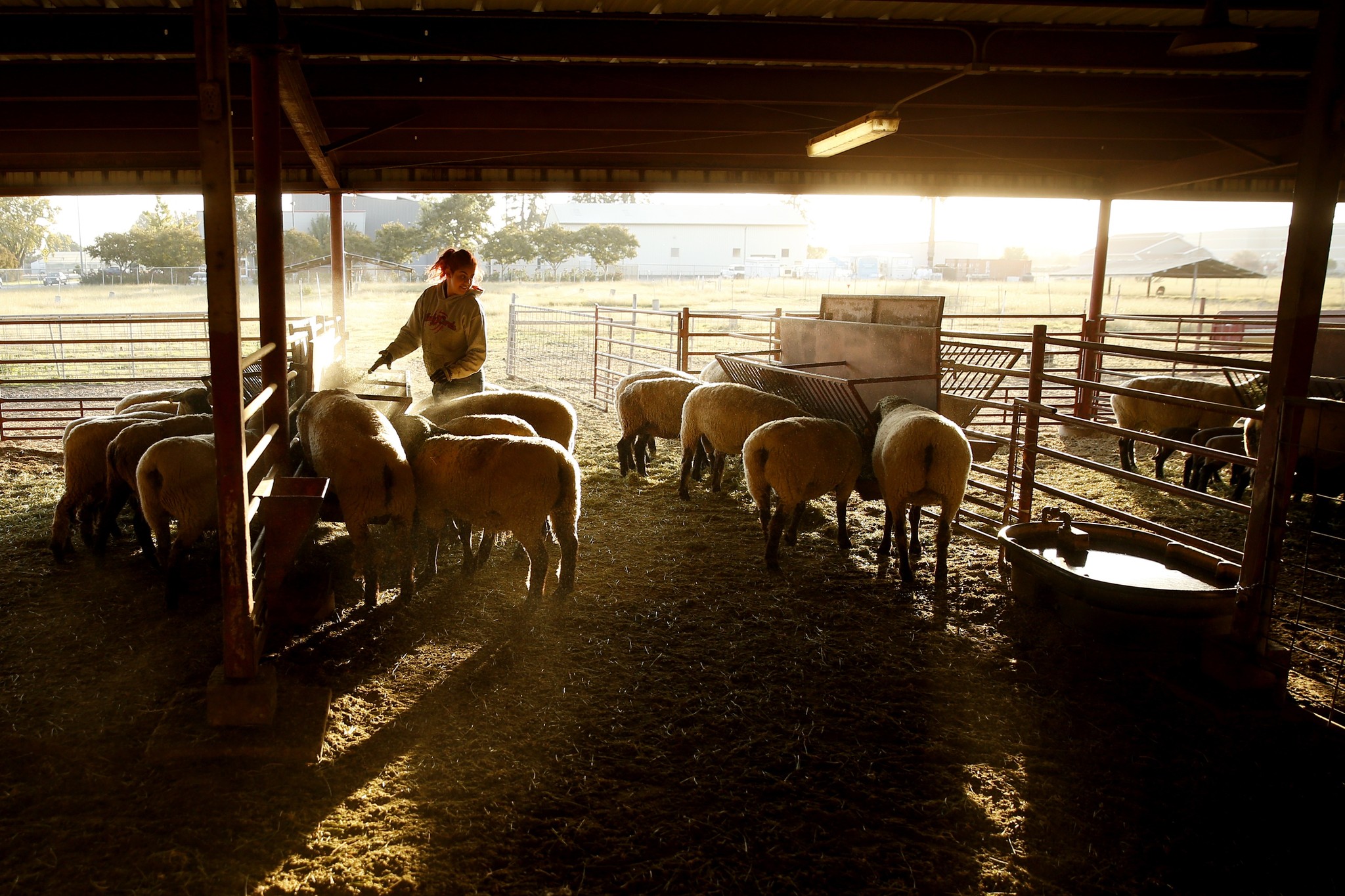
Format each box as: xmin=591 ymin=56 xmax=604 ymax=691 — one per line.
xmin=290 ymin=201 xmax=296 ymax=229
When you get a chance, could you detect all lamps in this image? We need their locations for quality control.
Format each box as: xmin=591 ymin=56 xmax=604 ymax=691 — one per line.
xmin=805 ymin=110 xmax=902 ymax=158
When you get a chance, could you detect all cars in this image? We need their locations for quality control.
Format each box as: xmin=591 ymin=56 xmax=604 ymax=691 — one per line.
xmin=0 ymin=277 xmax=3 ymax=289
xmin=188 ymin=272 xmax=207 ymax=286
xmin=43 ymin=272 xmax=67 ymax=286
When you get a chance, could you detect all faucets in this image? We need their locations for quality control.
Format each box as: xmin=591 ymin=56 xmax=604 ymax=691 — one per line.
xmin=1041 ymin=504 xmax=1073 ymax=528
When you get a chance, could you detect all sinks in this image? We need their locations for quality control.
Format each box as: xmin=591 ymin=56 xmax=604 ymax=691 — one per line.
xmin=997 ymin=521 xmax=1243 ymax=637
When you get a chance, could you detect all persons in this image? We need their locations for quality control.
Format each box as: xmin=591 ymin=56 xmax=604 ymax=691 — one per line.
xmin=368 ymin=248 xmax=488 ymax=405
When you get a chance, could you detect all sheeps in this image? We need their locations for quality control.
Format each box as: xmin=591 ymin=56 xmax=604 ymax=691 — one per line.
xmin=295 ymin=389 xmax=418 ymax=610
xmin=1152 ymin=425 xmax=1252 ymax=501
xmin=864 ymin=394 xmax=972 ymax=583
xmin=51 ymin=388 xmax=264 ymax=577
xmin=1110 ymin=368 xmax=1269 ymax=473
xmin=741 ymin=416 xmax=863 ymax=573
xmin=412 ymin=434 xmax=581 ymax=600
xmin=613 ymin=358 xmax=735 ymax=477
xmin=677 ymin=382 xmax=818 ymax=499
xmin=391 ymin=382 xmax=577 ymax=562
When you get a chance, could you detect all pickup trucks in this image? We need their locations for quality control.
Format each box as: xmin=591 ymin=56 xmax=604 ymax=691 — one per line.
xmin=720 ymin=265 xmax=746 ymax=279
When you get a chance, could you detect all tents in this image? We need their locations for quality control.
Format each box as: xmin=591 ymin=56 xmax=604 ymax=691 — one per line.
xmin=1147 ymin=258 xmax=1269 ymax=299
